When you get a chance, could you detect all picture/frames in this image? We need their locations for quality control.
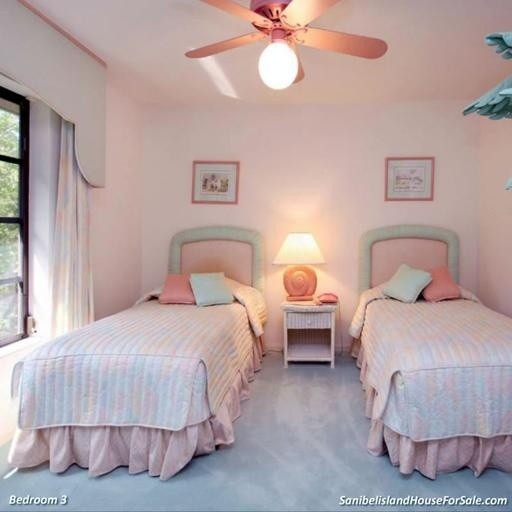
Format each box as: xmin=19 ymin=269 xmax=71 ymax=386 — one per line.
xmin=383 ymin=156 xmax=436 ymax=202
xmin=191 ymin=160 xmax=240 ymax=205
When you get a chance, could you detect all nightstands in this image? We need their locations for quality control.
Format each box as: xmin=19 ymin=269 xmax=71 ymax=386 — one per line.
xmin=280 ymin=301 xmax=338 ymax=370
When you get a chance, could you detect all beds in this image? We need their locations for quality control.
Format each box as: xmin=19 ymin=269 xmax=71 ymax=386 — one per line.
xmin=5 ymin=226 xmax=264 ymax=480
xmin=359 ymin=224 xmax=512 ymax=478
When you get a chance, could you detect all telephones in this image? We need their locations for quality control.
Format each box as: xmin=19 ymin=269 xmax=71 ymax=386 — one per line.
xmin=318 ymin=292 xmax=338 ymax=305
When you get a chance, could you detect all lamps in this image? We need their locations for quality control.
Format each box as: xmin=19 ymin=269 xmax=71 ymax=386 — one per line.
xmin=272 ymin=232 xmax=326 ymax=300
xmin=257 ymin=28 xmax=299 ymax=92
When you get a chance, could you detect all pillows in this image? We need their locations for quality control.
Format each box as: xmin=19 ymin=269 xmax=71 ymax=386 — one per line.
xmin=381 ymin=263 xmax=462 ymax=302
xmin=158 ymin=270 xmax=234 ymax=306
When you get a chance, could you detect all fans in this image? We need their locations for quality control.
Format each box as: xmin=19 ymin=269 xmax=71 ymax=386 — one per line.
xmin=183 ymin=1 xmax=388 ymax=87
